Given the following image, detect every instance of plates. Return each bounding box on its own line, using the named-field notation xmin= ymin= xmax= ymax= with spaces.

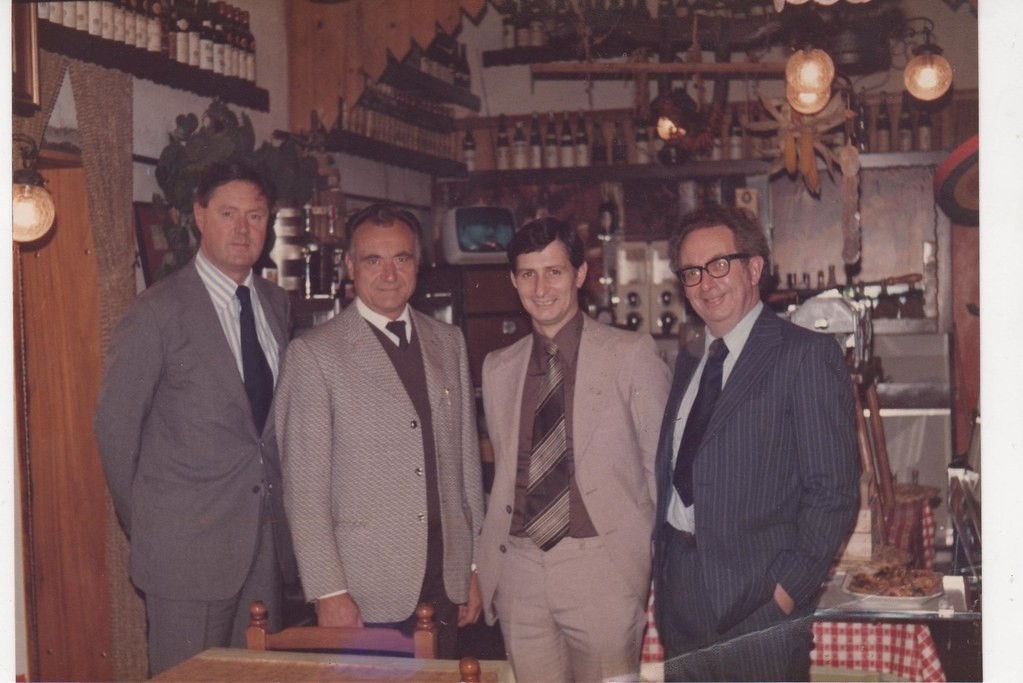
xmin=840 ymin=564 xmax=944 ymax=609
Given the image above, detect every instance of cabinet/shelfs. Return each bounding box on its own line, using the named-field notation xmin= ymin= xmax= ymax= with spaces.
xmin=432 ymin=148 xmax=955 ymax=393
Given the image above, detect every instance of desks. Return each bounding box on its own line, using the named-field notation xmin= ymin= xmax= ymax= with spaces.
xmin=152 ymin=646 xmax=516 ymax=683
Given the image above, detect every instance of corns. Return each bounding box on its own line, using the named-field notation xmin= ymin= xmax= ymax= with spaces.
xmin=782 ymin=126 xmax=821 ymax=194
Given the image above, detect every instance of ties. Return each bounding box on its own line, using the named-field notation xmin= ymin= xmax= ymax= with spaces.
xmin=235 ymin=286 xmax=276 ymax=435
xmin=385 ymin=321 xmax=409 ymax=352
xmin=673 ymin=338 xmax=729 ymax=508
xmin=522 ymin=342 xmax=570 ymax=552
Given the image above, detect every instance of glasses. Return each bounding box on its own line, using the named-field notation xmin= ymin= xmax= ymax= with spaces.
xmin=676 ymin=252 xmax=767 ymax=288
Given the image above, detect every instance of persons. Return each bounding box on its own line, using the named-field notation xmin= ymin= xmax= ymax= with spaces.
xmin=93 ymin=161 xmax=298 ymax=679
xmin=650 ymin=203 xmax=861 ymax=682
xmin=479 ymin=216 xmax=673 ymax=683
xmin=274 ymin=203 xmax=486 ymax=657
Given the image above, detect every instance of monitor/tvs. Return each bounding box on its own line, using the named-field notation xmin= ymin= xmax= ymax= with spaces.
xmin=443 ymin=206 xmax=520 ymax=266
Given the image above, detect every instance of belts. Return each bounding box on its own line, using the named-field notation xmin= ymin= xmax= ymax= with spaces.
xmin=668 ymin=521 xmax=696 ymax=546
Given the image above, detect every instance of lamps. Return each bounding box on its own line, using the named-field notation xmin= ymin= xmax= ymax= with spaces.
xmin=12 ymin=133 xmax=55 ymax=243
xmin=773 ymin=1 xmax=953 ymax=114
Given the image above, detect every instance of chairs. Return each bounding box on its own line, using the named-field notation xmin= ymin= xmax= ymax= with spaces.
xmin=241 ymin=600 xmax=439 ymax=656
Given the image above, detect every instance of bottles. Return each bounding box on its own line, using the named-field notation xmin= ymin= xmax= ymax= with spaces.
xmin=598 ymin=175 xmax=620 ymax=251
xmin=770 ymin=265 xmax=927 ymax=320
xmin=896 ymin=90 xmax=913 ymax=151
xmin=513 ymin=120 xmax=527 ymax=170
xmin=299 ymin=202 xmax=322 ymax=300
xmin=876 ymin=91 xmax=890 ymax=153
xmin=495 ymin=114 xmax=510 ymax=169
xmin=321 ymin=203 xmax=345 ymax=298
xmin=462 ymin=125 xmax=476 ymax=173
xmin=854 ymin=100 xmax=868 ymax=153
xmin=528 ymin=103 xmax=780 ymax=171
xmin=918 ymin=102 xmax=931 ymax=150
xmin=36 ymin=0 xmax=255 ymax=82
